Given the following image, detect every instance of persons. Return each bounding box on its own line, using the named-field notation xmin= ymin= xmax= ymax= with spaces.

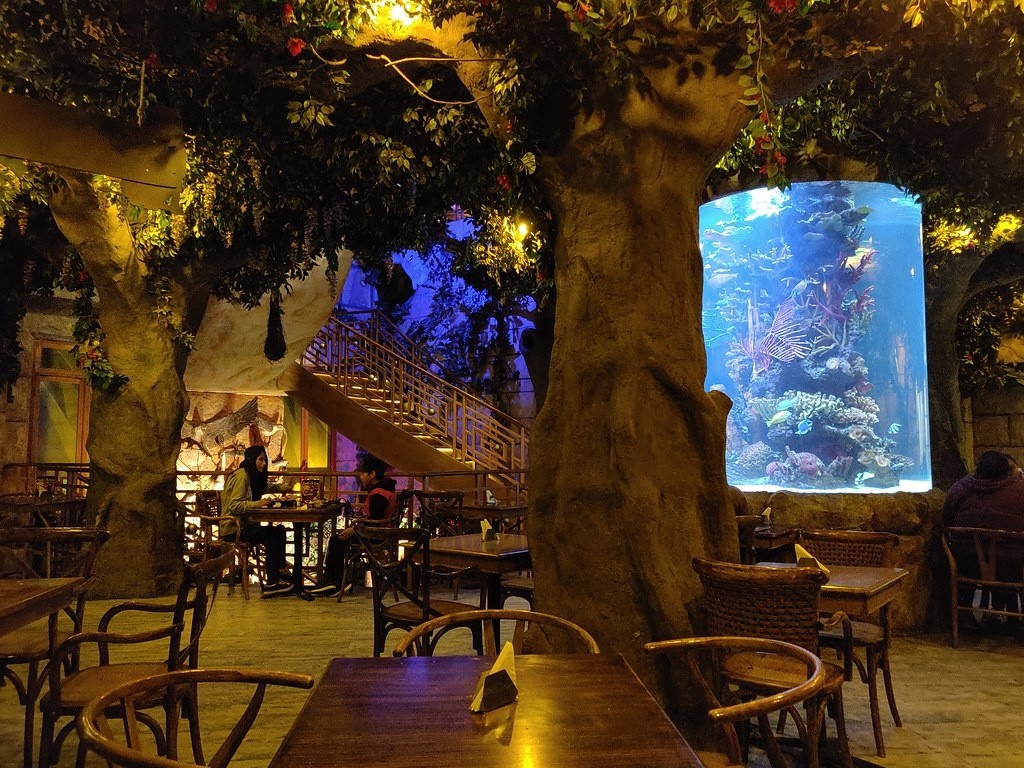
xmin=310 ymin=456 xmax=401 ymax=598
xmin=219 ymin=444 xmax=295 ymax=596
xmin=938 ymin=450 xmax=1024 ymax=644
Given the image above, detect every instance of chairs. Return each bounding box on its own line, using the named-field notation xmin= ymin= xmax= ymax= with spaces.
xmin=500 ymin=576 xmax=535 ymax=611
xmin=796 ymin=530 xmax=902 ymax=758
xmin=691 ymin=556 xmax=854 ymax=768
xmin=0 ymin=527 xmax=111 ymax=768
xmin=38 ymin=542 xmax=235 ymax=768
xmin=644 ymin=636 xmax=827 ymax=768
xmin=736 ymin=515 xmax=762 ymax=565
xmin=338 ymin=490 xmax=408 ymax=602
xmin=76 ymin=667 xmax=313 ymax=768
xmin=941 ymin=526 xmax=1024 ymax=648
xmin=196 ymin=491 xmax=264 ymax=600
xmin=354 ymin=523 xmax=484 ymax=656
xmin=414 ymin=489 xmax=465 ymax=599
xmin=393 ymin=609 xmax=600 ymax=657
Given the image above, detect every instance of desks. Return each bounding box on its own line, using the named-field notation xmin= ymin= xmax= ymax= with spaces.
xmin=753 ymin=562 xmax=910 ymax=768
xmin=247 ymin=500 xmax=344 ymax=601
xmin=398 ymin=533 xmax=532 ymax=655
xmin=268 ymin=655 xmax=707 ymax=768
xmin=0 ymin=497 xmax=86 ymax=578
xmin=0 ymin=578 xmax=90 ymax=674
xmin=453 ymin=506 xmax=528 ymax=535
xmin=739 ymin=524 xmax=800 ymax=565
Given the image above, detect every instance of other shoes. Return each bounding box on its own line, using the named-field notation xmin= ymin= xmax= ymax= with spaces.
xmin=959 ymin=614 xmax=980 ymax=631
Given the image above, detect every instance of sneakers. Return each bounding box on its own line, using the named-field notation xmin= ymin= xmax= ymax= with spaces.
xmin=311 ymin=578 xmax=336 ymax=593
xmin=264 ymin=581 xmax=294 ymax=595
xmin=329 ymin=581 xmax=352 ymax=596
xmin=279 ymin=572 xmax=294 ymax=582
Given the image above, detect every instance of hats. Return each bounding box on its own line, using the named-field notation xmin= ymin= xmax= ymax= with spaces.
xmin=358 ymin=455 xmax=386 ymax=471
